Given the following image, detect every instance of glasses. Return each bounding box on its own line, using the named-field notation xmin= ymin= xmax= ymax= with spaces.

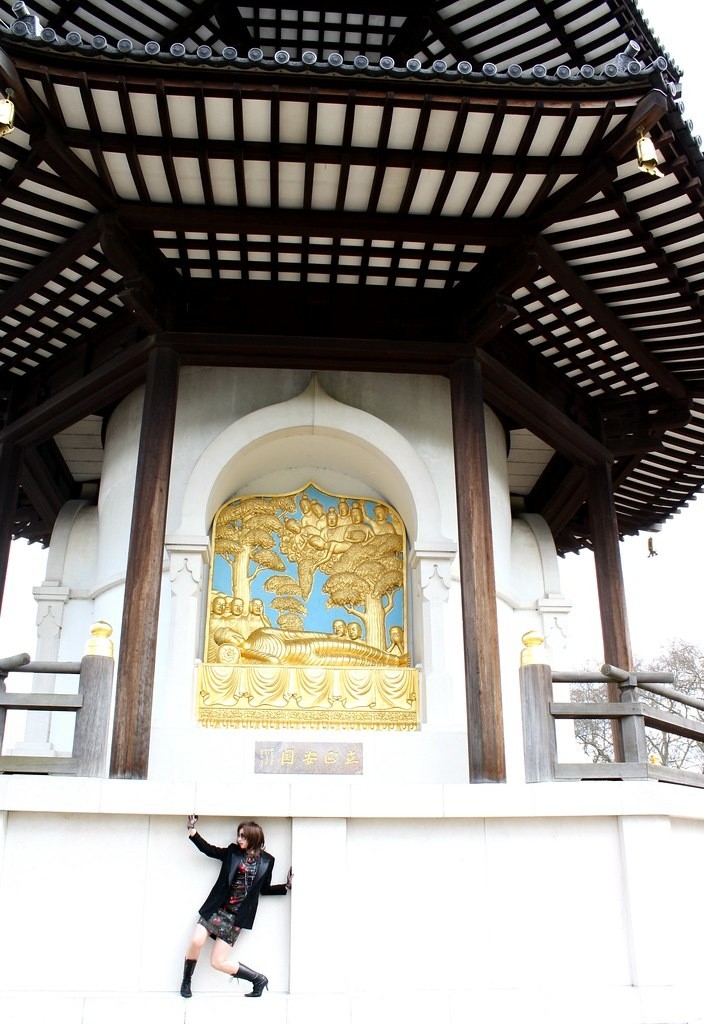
xmin=237 ymin=834 xmax=248 ymax=840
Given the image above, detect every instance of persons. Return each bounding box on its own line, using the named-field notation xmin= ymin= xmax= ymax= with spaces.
xmin=180 ymin=815 xmax=293 ymax=998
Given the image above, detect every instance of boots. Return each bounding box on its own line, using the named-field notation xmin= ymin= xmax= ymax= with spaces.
xmin=230 ymin=961 xmax=268 ymax=997
xmin=180 ymin=957 xmax=197 ymax=998
